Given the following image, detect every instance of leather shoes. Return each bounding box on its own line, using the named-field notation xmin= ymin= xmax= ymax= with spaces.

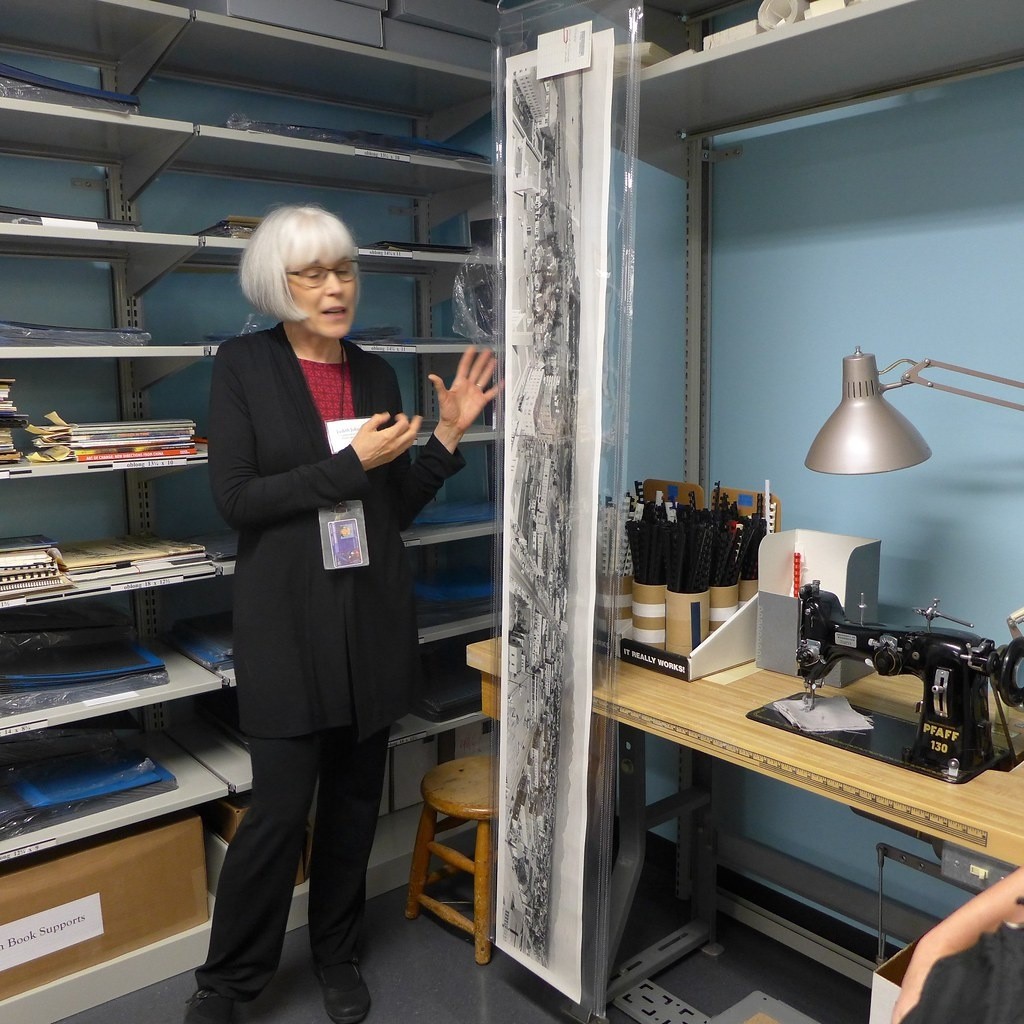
xmin=317 ymin=962 xmax=371 ymax=1024
xmin=184 ymin=989 xmax=235 ymax=1024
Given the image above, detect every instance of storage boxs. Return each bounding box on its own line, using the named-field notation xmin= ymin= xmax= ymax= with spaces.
xmin=757 ymin=528 xmax=881 ymax=688
xmin=0 ymin=809 xmax=209 ymax=1001
xmin=869 ymin=936 xmax=919 ymax=1024
xmin=619 ymin=479 xmax=782 ymax=682
xmin=211 ymin=718 xmax=493 ymax=886
xmin=178 ymin=0 xmax=523 ymax=76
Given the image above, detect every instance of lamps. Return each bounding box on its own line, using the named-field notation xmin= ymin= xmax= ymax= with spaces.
xmin=804 ymin=346 xmax=1024 ymax=475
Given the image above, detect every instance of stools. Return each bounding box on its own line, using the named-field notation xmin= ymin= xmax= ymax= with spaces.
xmin=405 ymin=754 xmax=499 ymax=964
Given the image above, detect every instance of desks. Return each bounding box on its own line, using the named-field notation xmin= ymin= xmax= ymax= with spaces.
xmin=468 ymin=635 xmax=1024 ymax=1024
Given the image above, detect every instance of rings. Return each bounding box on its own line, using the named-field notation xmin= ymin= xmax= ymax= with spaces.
xmin=477 ymin=384 xmax=484 ymax=390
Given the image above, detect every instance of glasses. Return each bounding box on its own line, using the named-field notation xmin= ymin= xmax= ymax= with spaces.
xmin=286 ymin=259 xmax=360 ymax=288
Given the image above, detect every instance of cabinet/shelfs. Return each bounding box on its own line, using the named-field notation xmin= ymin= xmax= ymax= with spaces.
xmin=0 ymin=0 xmax=515 ymax=1024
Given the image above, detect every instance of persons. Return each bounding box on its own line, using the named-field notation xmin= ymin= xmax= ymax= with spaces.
xmin=184 ymin=205 xmax=502 ymax=1024
xmin=890 ymin=865 xmax=1024 ymax=1024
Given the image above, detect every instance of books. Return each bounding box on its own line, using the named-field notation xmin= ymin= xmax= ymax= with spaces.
xmin=0 ymin=207 xmax=262 ymax=599
